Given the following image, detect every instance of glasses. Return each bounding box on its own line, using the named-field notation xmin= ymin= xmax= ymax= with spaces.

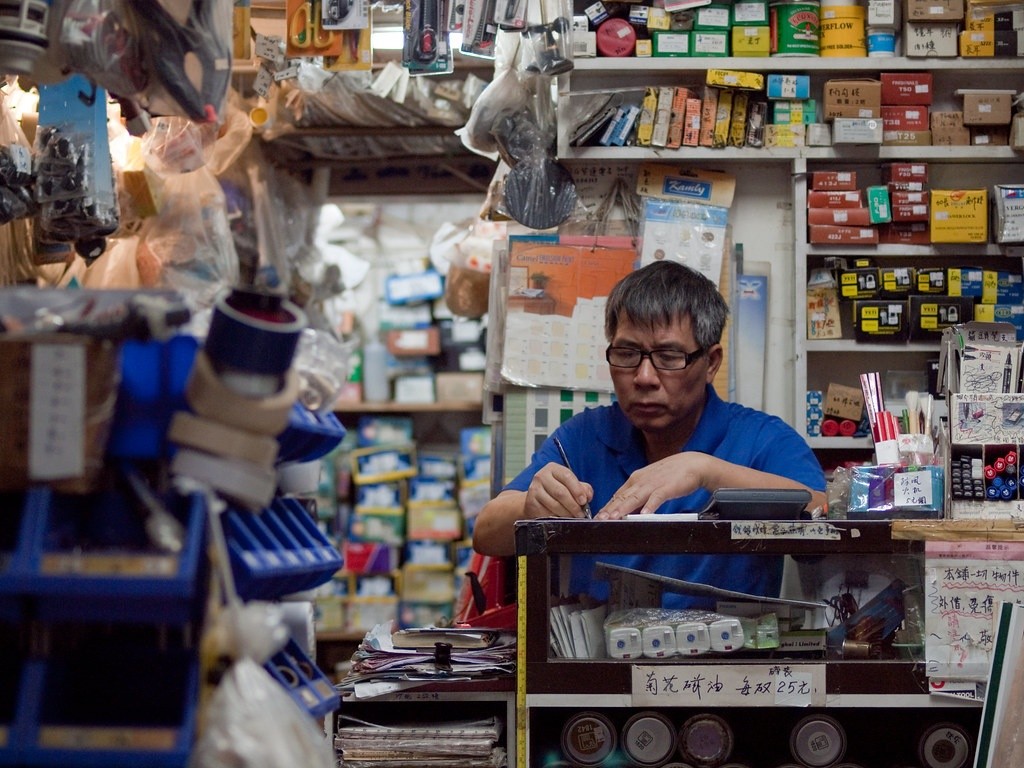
xmin=606 ymin=343 xmax=705 ymax=370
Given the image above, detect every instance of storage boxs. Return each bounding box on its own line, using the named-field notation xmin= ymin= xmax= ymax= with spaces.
xmin=731 ymin=0 xmax=770 ymax=26
xmin=691 ymin=31 xmax=728 ymax=57
xmin=636 ymin=40 xmax=653 ymax=57
xmin=647 ymin=7 xmax=672 ymax=38
xmin=732 ymin=26 xmax=770 ymax=57
xmin=806 ymin=258 xmax=1024 ymax=520
xmin=807 ymin=163 xmax=1024 ymax=245
xmin=584 ymin=1 xmax=628 ymax=26
xmin=327 ymin=249 xmax=490 ymax=405
xmin=283 ymin=416 xmax=493 ymax=635
xmin=629 ymin=5 xmax=649 ymax=25
xmin=573 ymin=14 xmax=588 ymax=32
xmin=867 ymin=0 xmax=1024 ymax=59
xmin=574 ymin=31 xmax=597 ymax=58
xmin=653 ymin=31 xmax=691 ymax=57
xmin=694 ymin=4 xmax=732 ymax=30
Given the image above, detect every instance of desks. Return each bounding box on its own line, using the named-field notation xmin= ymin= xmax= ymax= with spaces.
xmin=510 ymin=517 xmax=1024 ymax=768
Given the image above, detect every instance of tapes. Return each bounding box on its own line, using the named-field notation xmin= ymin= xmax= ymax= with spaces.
xmin=166 ymin=409 xmax=280 ymax=465
xmin=187 ymin=348 xmax=301 ymax=431
xmin=205 ymin=295 xmax=308 ymax=376
xmin=172 ymin=446 xmax=277 ymax=509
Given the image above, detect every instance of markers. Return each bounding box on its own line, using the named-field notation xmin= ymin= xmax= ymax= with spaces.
xmin=984 ymin=451 xmax=1024 ymax=500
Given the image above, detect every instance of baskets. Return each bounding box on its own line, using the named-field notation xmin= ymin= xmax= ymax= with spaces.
xmin=0 ymin=332 xmax=122 ymax=493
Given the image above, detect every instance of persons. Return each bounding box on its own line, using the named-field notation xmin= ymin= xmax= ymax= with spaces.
xmin=473 ymin=261 xmax=829 ymax=614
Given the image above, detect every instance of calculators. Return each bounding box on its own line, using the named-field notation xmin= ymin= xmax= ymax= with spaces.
xmin=698 ymin=487 xmax=812 ymax=519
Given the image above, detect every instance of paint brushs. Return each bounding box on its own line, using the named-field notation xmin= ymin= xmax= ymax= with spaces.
xmin=904 ymin=390 xmax=933 ymax=436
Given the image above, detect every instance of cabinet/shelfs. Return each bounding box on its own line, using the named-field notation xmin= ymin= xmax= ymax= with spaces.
xmin=556 ymin=56 xmax=1024 ymax=450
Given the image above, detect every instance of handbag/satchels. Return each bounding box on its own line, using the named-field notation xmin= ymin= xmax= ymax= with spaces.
xmin=191 ymin=599 xmax=335 ymax=768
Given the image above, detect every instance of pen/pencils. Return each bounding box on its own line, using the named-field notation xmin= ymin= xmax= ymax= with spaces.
xmin=962 ymin=403 xmax=970 ymax=421
xmin=554 ymin=437 xmax=594 ymax=520
xmin=1002 ymin=350 xmax=1012 ymax=393
